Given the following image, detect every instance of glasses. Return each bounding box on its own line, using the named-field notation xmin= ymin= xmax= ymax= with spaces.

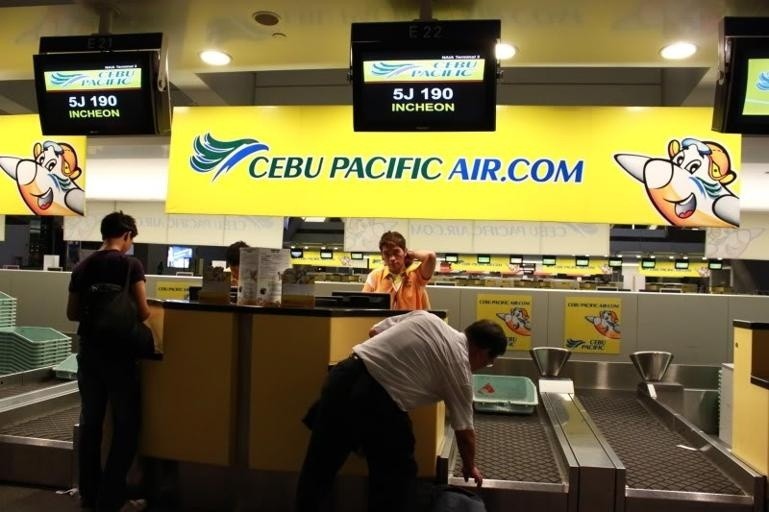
xmin=483 ymin=361 xmax=495 ymax=367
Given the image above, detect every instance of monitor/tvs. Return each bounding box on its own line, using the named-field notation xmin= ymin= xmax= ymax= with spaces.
xmin=166 ymin=245 xmax=196 ymax=273
xmin=351 ymin=37 xmax=496 ymax=132
xmin=711 ymin=36 xmax=769 ymax=136
xmin=332 ymin=292 xmax=391 ymax=310
xmin=32 ymin=51 xmax=170 ymax=135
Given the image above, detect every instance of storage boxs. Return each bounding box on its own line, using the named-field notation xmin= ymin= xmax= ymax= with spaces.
xmin=237 ymin=248 xmax=290 ymax=305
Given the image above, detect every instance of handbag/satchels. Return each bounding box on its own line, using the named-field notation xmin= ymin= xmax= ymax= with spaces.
xmin=104 ymin=255 xmax=138 ymax=335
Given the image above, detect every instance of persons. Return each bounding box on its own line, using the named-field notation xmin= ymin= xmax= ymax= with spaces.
xmin=223 ymin=239 xmax=252 ymax=288
xmin=65 ymin=211 xmax=156 ymax=509
xmin=362 ymin=233 xmax=436 ymax=312
xmin=285 ymin=309 xmax=506 ymax=511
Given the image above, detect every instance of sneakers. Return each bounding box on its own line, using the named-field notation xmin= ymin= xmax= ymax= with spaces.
xmin=120 ymin=498 xmax=147 ymax=512
